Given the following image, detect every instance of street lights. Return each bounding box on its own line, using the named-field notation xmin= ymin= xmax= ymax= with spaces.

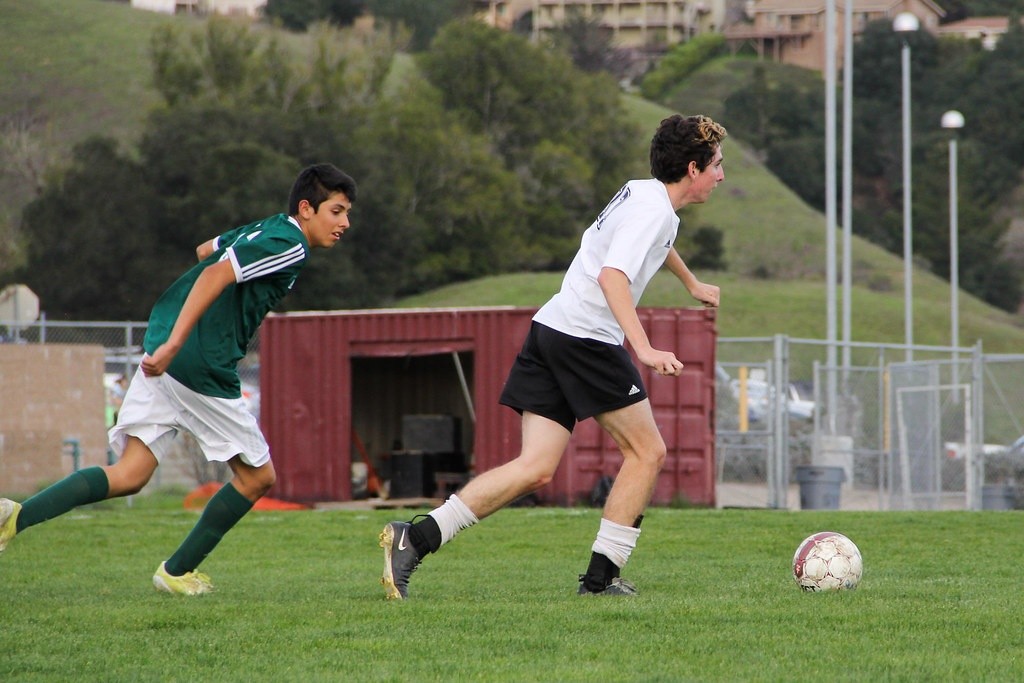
xmin=889 ymin=8 xmax=922 ymax=360
xmin=941 ymin=109 xmax=968 ymax=349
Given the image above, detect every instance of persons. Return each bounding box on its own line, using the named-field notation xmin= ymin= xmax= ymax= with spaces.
xmin=2 ymin=162 xmax=359 ymax=599
xmin=378 ymin=112 xmax=730 ymax=605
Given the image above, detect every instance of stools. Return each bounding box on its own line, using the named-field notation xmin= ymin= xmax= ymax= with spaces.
xmin=435 ymin=472 xmax=467 ymax=496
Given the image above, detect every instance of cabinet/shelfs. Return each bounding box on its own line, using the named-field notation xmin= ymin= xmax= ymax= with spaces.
xmin=400 ymin=414 xmax=464 ymax=453
xmin=389 ymin=450 xmax=449 ymax=498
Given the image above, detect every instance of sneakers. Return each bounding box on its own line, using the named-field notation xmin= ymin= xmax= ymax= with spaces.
xmin=152 ymin=560 xmax=214 ymax=596
xmin=0 ymin=497 xmax=23 ymax=551
xmin=576 ymin=577 xmax=641 ymax=596
xmin=378 ymin=521 xmax=423 ymax=600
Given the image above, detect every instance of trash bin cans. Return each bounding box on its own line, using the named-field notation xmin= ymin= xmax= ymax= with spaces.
xmin=792 ymin=464 xmax=848 ymax=510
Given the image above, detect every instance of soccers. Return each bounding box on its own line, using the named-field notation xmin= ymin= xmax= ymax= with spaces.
xmin=792 ymin=531 xmax=864 ymax=593
